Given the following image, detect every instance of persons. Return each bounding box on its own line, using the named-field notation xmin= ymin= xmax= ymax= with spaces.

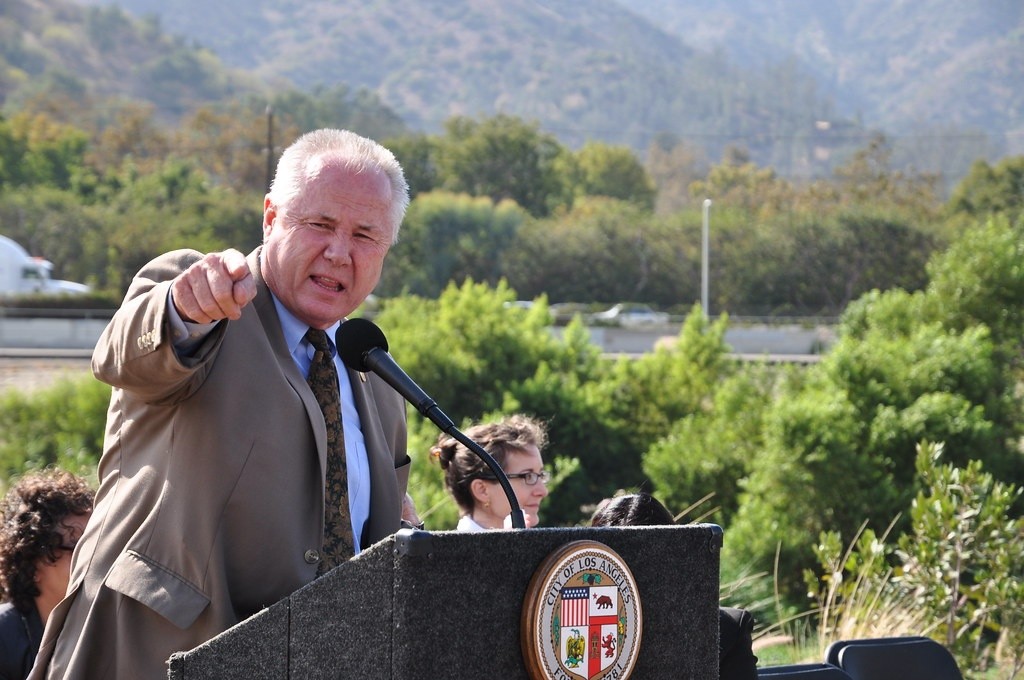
xmin=401 ymin=491 xmax=425 ymax=531
xmin=27 ymin=128 xmax=412 ymax=680
xmin=590 ymin=483 xmax=759 ymax=680
xmin=429 ymin=410 xmax=551 ymax=532
xmin=1 ymin=462 xmax=96 ymax=680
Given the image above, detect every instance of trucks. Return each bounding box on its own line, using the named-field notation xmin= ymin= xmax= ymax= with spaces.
xmin=0 ymin=231 xmax=92 ymax=300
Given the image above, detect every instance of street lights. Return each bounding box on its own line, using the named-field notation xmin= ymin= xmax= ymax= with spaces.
xmin=699 ymin=196 xmax=712 ymax=319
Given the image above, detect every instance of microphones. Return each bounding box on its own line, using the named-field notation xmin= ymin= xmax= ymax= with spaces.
xmin=334 ymin=318 xmax=528 ymax=527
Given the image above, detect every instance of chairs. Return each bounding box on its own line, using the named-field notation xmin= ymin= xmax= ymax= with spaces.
xmin=756 ymin=634 xmax=962 ymax=680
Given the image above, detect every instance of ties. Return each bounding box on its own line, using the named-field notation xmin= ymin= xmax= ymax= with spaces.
xmin=304 ymin=327 xmax=355 ymax=581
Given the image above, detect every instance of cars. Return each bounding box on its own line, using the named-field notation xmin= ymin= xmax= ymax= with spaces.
xmin=548 ymin=301 xmax=590 ymax=325
xmin=591 ymin=300 xmax=670 ymax=329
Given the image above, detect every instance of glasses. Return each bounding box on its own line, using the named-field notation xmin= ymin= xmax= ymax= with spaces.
xmin=481 ymin=469 xmax=551 ymax=485
